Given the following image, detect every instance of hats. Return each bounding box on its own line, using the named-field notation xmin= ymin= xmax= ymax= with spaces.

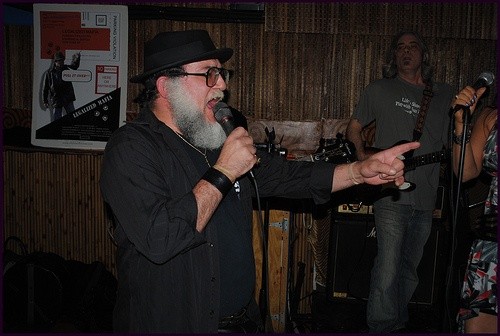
xmin=129 ymin=28 xmax=234 ymax=83
xmin=52 ymin=52 xmax=65 ymax=62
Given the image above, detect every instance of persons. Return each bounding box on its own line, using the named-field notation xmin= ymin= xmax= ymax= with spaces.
xmin=449 ymin=86 xmax=500 ymax=336
xmin=346 ymin=30 xmax=454 ymax=336
xmin=99 ymin=30 xmax=421 ymax=336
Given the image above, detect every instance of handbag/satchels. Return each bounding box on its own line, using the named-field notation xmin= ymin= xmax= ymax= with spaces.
xmin=5 ymin=236 xmax=117 ymax=336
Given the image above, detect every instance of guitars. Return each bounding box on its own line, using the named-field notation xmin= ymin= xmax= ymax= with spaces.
xmin=350 ymin=140 xmax=451 ymax=205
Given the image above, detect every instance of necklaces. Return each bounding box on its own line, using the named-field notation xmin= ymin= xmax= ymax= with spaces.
xmin=171 ymin=126 xmax=209 ymax=166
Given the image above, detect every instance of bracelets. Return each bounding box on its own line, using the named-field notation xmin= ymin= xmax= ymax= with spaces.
xmin=453 ymin=130 xmax=471 ymax=145
xmin=348 ymin=160 xmax=365 ymax=185
xmin=200 ymin=167 xmax=233 ymax=197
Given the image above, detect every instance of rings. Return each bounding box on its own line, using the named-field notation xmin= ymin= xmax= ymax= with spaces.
xmin=455 ymin=94 xmax=459 ymax=99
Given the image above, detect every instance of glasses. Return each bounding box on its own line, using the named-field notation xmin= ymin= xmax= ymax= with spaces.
xmin=174 ymin=66 xmax=235 ymax=87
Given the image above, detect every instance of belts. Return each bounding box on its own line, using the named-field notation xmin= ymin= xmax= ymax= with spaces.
xmin=218 ymin=306 xmax=247 ymax=328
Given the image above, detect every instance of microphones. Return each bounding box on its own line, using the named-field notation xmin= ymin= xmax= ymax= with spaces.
xmin=452 ymin=71 xmax=496 ymax=113
xmin=212 ymin=102 xmax=255 ymax=178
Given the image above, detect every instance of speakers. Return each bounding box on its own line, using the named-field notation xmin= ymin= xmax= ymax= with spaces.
xmin=326 ymin=215 xmax=469 ymax=336
